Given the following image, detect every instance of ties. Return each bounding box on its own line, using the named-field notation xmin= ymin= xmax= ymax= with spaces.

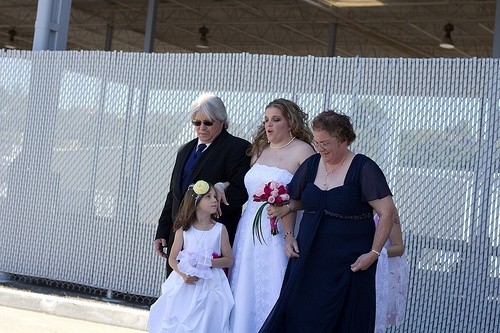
xmin=193 ymin=144 xmax=207 ymax=160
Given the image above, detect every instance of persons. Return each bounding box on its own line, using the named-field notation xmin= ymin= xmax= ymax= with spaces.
xmin=155 ymin=92 xmax=252 ymax=278
xmin=374 ymin=206 xmax=408 ymax=333
xmin=258 ymin=111 xmax=394 ymax=333
xmin=148 ymin=181 xmax=235 ymax=333
xmin=214 ymin=98 xmax=317 ymax=333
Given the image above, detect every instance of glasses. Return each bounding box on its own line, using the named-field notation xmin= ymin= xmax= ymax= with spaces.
xmin=313 ymin=137 xmax=340 ymax=148
xmin=191 ymin=119 xmax=214 ymax=126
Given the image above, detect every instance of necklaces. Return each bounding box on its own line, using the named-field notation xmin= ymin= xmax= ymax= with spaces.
xmin=324 ymin=150 xmax=349 ymax=186
xmin=270 ymin=137 xmax=295 ymax=149
xmin=194 ymin=232 xmax=204 ymax=243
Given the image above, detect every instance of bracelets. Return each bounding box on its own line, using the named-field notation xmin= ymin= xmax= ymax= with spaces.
xmin=284 ymin=232 xmax=291 ymax=239
xmin=287 ymin=204 xmax=291 ymax=211
xmin=372 ymin=250 xmax=379 ymax=255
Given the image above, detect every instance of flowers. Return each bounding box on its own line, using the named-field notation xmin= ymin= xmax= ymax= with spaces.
xmin=253 ymin=181 xmax=290 ymax=246
xmin=193 ymin=180 xmax=209 ymax=194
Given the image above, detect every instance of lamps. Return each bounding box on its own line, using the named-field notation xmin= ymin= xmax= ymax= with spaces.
xmin=197 ymin=26 xmax=209 ymax=48
xmin=439 ymin=23 xmax=456 ymax=49
xmin=5 ymin=27 xmax=17 ymax=48
